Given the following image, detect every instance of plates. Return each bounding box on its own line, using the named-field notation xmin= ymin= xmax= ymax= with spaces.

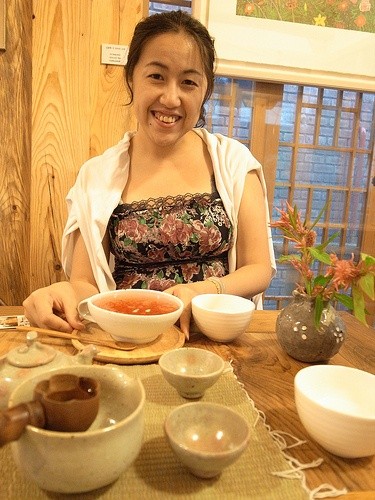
xmin=70 ymin=319 xmax=185 ymax=364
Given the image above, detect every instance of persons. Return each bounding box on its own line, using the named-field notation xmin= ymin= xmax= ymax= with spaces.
xmin=22 ymin=9 xmax=276 ymax=341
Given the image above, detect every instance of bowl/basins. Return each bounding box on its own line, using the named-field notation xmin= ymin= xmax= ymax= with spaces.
xmin=191 ymin=293 xmax=255 ymax=343
xmin=158 ymin=347 xmax=225 ymax=399
xmin=295 ymin=364 xmax=375 ymax=458
xmin=77 ymin=289 xmax=184 ymax=344
xmin=164 ymin=401 xmax=253 ymax=480
xmin=8 ymin=363 xmax=146 ymax=494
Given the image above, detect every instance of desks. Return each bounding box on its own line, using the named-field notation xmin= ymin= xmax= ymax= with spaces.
xmin=0 ymin=305 xmax=375 ymax=500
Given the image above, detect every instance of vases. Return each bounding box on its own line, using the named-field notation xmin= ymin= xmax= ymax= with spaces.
xmin=276 ymin=290 xmax=345 ymax=362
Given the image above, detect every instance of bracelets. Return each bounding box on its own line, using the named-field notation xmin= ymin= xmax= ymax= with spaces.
xmin=206 ymin=277 xmax=223 ymax=294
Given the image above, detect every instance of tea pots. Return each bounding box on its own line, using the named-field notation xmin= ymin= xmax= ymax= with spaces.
xmin=0 ymin=331 xmax=100 ymax=407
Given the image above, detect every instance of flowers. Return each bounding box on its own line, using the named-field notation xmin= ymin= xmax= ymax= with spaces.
xmin=268 ymin=200 xmax=375 ymax=333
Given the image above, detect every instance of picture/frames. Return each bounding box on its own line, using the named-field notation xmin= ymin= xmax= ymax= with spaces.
xmin=190 ymin=0 xmax=375 ymax=94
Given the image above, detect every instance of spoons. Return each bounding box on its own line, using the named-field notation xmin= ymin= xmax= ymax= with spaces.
xmin=0 ymin=373 xmax=101 ymax=448
xmin=16 ymin=326 xmax=139 ymax=350
